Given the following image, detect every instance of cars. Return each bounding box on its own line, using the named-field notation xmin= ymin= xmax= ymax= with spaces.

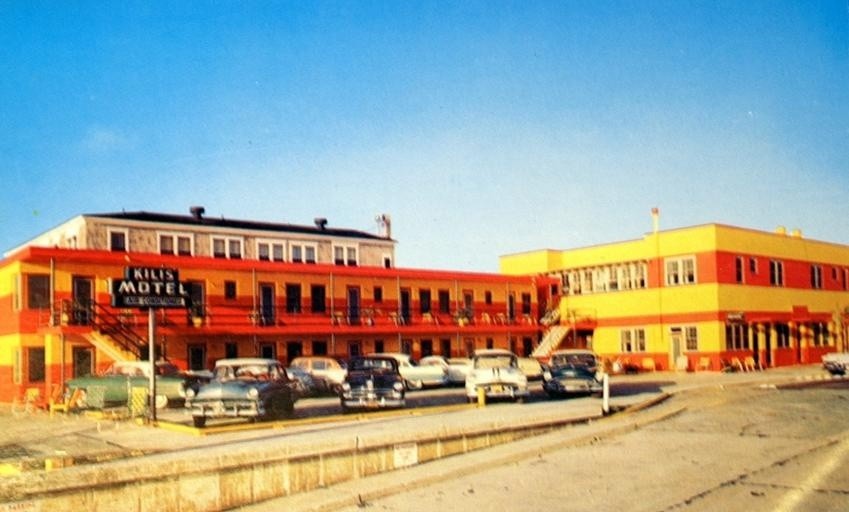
xmin=822 ymin=350 xmax=849 ymax=376
